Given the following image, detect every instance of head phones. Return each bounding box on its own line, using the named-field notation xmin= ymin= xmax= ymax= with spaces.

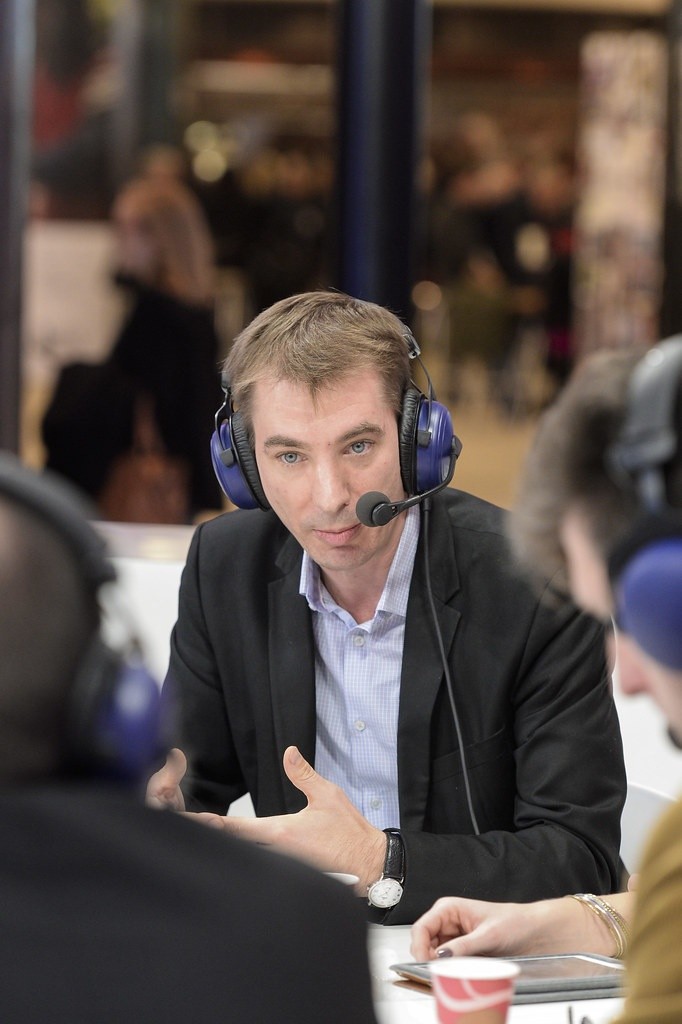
xmin=615 ymin=334 xmax=682 ymax=674
xmin=206 ymin=331 xmax=460 ymax=514
xmin=0 ymin=458 xmax=170 ymax=807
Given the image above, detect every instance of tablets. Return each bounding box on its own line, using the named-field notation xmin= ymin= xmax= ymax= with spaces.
xmin=390 ymin=951 xmax=627 ymax=994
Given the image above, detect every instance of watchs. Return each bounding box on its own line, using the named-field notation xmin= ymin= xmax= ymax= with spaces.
xmin=366 ymin=832 xmax=405 ymax=924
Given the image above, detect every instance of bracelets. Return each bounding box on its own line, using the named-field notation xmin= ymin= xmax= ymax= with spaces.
xmin=566 ymin=894 xmax=633 ymax=959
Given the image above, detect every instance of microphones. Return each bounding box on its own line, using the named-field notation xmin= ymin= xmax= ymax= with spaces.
xmin=355 ymin=454 xmax=457 ymax=528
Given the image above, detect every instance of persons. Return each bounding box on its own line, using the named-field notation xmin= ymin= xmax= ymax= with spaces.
xmin=139 ymin=292 xmax=630 ymax=926
xmin=411 ymin=334 xmax=682 ymax=1024
xmin=444 ymin=105 xmax=571 ymax=286
xmin=208 ymin=118 xmax=330 ymax=309
xmin=96 ymin=172 xmax=232 ymax=525
xmin=0 ymin=447 xmax=376 ymax=1024
xmin=36 ymin=83 xmax=123 ymax=194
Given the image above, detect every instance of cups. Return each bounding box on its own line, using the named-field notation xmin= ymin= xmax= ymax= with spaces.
xmin=321 ymin=873 xmax=360 ymax=893
xmin=430 ymin=956 xmax=520 ymax=1024
